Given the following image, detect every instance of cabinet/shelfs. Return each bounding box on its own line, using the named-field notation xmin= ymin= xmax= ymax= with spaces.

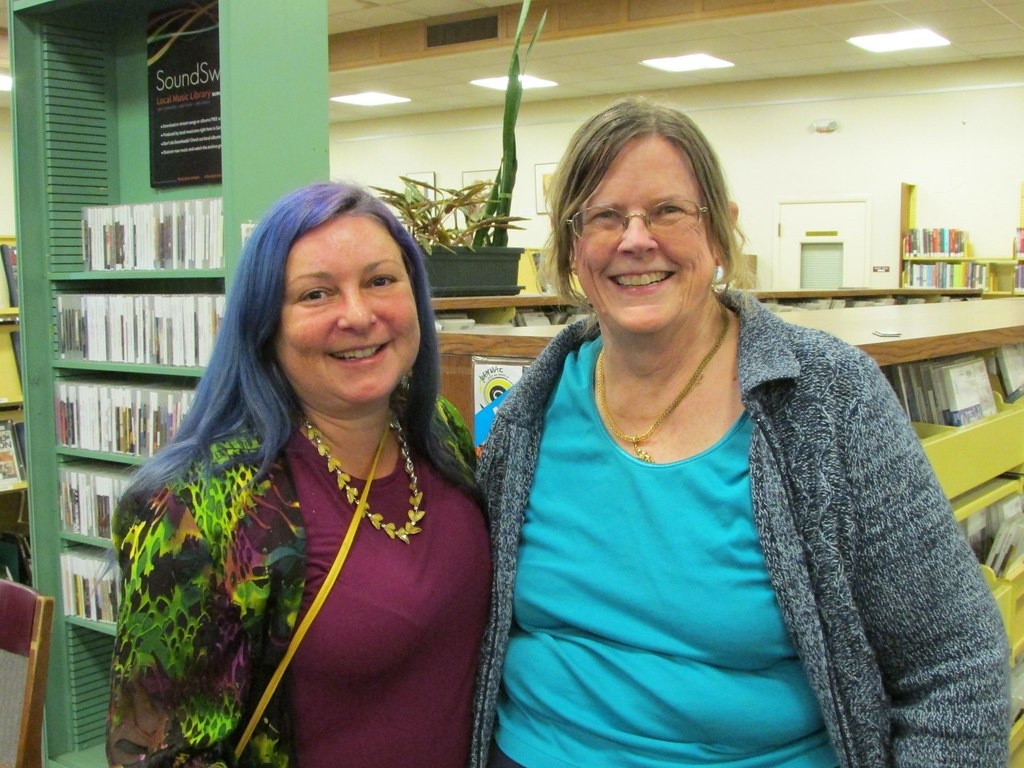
xmin=430 ymin=291 xmax=1021 ymax=767
xmin=10 ymin=1 xmax=329 ymax=768
xmin=897 ymin=183 xmax=1024 ymax=292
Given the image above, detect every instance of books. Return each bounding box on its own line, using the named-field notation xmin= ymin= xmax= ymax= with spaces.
xmin=761 ymin=226 xmax=1024 ymax=729
xmin=0 ymin=194 xmax=225 ymax=630
xmin=430 ymin=303 xmax=594 ymax=329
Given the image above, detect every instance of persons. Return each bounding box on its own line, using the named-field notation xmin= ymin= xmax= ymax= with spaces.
xmin=474 ymin=98 xmax=1014 ymax=768
xmin=104 ymin=185 xmax=490 ymax=768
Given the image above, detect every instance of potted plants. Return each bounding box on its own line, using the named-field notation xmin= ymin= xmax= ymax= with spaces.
xmin=416 ymin=0 xmax=550 ymax=294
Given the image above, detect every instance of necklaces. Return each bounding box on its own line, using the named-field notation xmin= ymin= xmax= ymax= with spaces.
xmin=596 ymin=301 xmax=731 ymax=465
xmin=296 ymin=409 xmax=426 ymax=546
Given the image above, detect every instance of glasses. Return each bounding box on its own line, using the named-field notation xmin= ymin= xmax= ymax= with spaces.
xmin=567 ymin=197 xmax=708 ymax=244
